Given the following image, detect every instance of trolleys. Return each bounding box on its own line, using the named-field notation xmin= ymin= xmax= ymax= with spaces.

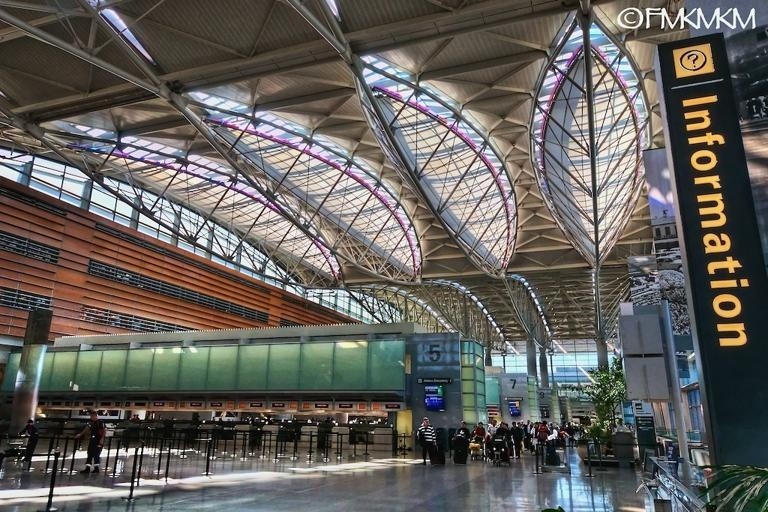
xmin=5 ymin=432 xmax=29 ymax=464
xmin=468 ymin=439 xmax=485 ymax=462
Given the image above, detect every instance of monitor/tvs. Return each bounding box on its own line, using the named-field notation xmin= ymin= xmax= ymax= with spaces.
xmin=540 ymin=406 xmax=549 ymax=418
xmin=424 ymin=384 xmax=446 ymax=412
xmin=508 ymin=400 xmax=521 ymax=417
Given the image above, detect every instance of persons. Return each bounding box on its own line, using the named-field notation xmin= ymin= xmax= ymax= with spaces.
xmin=451 ymin=418 xmax=581 ymax=466
xmin=17 ymin=473 xmax=32 ymax=490
xmin=75 ymin=409 xmax=105 ymax=475
xmin=75 ymin=476 xmax=98 ymax=511
xmin=416 ymin=417 xmax=438 ymax=465
xmin=19 ymin=419 xmax=39 ymax=471
